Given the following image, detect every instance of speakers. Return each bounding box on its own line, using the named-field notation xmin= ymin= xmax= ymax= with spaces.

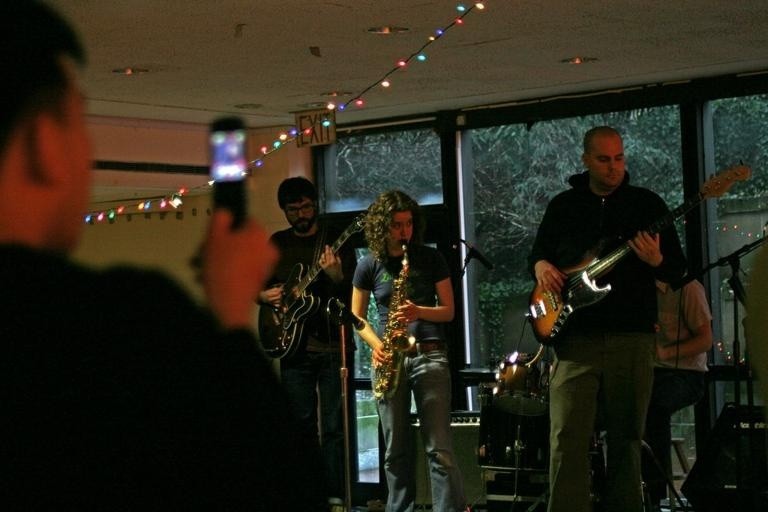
xmin=681 ymin=403 xmax=768 ymax=512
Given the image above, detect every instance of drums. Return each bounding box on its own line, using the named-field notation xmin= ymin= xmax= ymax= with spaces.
xmin=493 ymin=352 xmax=549 ymax=417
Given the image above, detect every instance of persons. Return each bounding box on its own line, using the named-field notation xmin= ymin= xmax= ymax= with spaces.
xmin=527 ymin=125 xmax=687 ymax=511
xmin=0 ymin=1 xmax=338 ymax=511
xmin=348 ymin=187 xmax=467 ymax=511
xmin=254 ymin=175 xmax=357 ymax=506
xmin=641 ymin=275 xmax=714 ymax=498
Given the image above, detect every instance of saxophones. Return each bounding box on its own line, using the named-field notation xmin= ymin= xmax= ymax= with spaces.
xmin=370 ymin=244 xmax=411 ymax=400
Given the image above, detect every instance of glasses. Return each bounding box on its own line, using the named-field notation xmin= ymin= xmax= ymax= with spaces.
xmin=283 ymin=202 xmax=316 ymax=217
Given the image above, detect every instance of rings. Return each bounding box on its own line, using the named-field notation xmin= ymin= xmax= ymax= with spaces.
xmin=322 ymin=260 xmax=326 ymax=264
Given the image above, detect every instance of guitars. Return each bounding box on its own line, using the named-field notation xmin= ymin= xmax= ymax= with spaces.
xmin=259 ymin=214 xmax=367 ymax=359
xmin=529 ymin=165 xmax=751 ymax=343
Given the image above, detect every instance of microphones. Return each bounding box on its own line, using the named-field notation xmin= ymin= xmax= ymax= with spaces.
xmin=462 ymin=240 xmax=493 ymax=271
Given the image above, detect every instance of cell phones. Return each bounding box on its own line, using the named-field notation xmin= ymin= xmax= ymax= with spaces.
xmin=207 ymin=114 xmax=250 ymax=233
